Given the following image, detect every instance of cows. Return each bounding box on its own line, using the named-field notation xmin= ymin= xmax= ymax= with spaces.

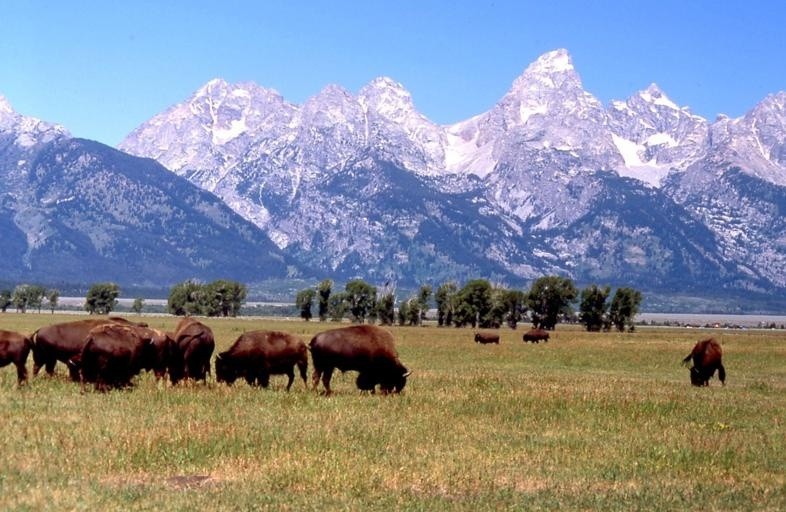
xmin=305 ymin=324 xmax=413 ymax=397
xmin=30 ymin=314 xmax=215 ymax=396
xmin=475 ymin=333 xmax=499 ymax=344
xmin=523 ymin=330 xmax=550 ymax=344
xmin=215 ymin=330 xmax=308 ymax=393
xmin=0 ymin=330 xmax=31 ymax=390
xmin=680 ymin=337 xmax=725 ymax=387
